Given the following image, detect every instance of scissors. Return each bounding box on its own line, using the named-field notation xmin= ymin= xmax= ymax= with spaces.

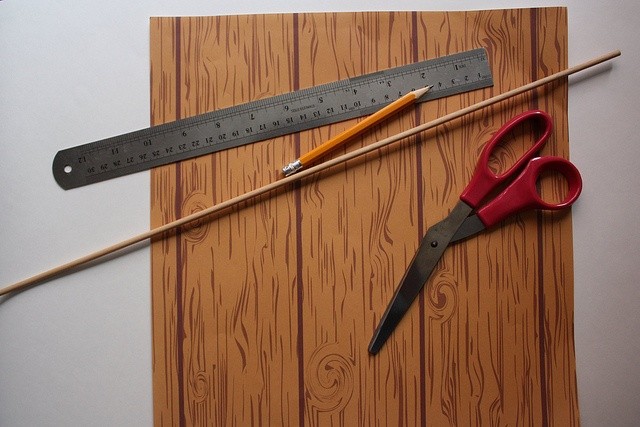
xmin=368 ymin=109 xmax=583 ymax=356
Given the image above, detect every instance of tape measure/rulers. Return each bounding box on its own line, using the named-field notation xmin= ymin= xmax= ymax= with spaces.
xmin=52 ymin=49 xmax=494 ymax=190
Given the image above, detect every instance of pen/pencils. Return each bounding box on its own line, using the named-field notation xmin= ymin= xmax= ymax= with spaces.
xmin=282 ymin=82 xmax=434 ymax=176
